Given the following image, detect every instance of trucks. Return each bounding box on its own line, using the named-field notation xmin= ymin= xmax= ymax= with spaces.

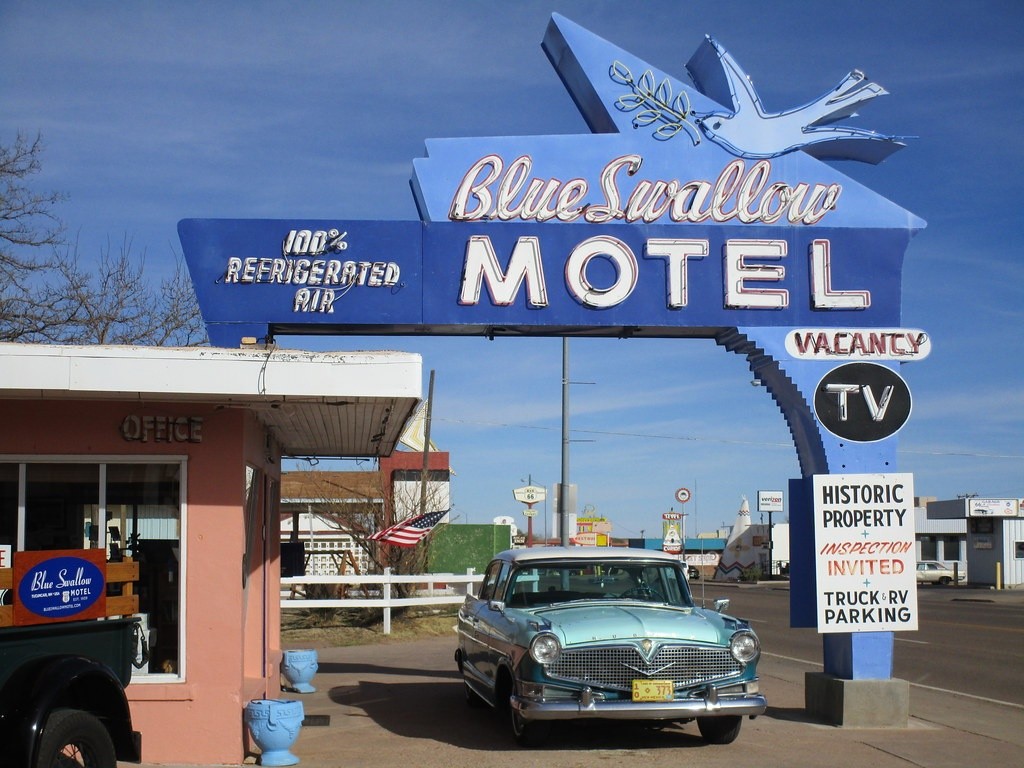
xmin=0 ymin=548 xmax=149 ymax=768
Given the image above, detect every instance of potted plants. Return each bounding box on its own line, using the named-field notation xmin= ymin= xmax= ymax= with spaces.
xmin=244 ymin=699 xmax=306 ymax=767
xmin=281 ymin=649 xmax=319 ymax=694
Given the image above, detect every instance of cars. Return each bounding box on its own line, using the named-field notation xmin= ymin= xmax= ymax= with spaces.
xmin=916 ymin=560 xmax=966 ymax=585
xmin=666 ymin=561 xmax=700 ymax=582
xmin=454 ymin=545 xmax=768 ymax=746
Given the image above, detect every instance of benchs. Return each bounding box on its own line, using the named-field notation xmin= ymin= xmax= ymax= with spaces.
xmin=512 ymin=590 xmax=605 ymax=606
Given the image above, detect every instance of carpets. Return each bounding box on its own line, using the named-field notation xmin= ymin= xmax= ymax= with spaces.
xmin=302 ymin=714 xmax=330 ymax=726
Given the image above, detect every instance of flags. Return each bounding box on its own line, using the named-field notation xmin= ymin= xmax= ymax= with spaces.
xmin=365 ymin=508 xmax=450 ymax=548
